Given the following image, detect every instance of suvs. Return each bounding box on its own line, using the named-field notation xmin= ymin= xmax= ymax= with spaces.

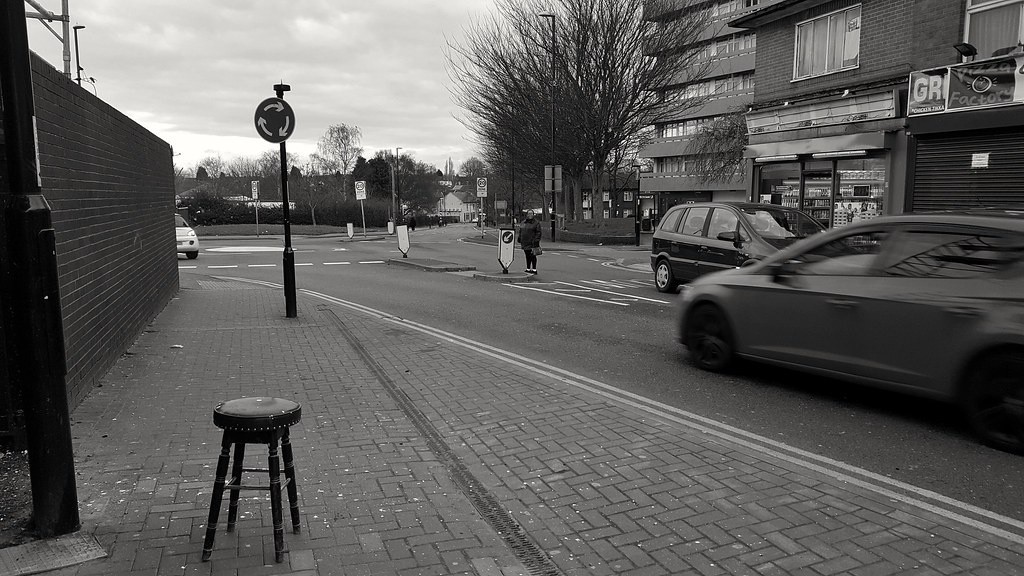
xmin=650 ymin=198 xmax=859 ymax=293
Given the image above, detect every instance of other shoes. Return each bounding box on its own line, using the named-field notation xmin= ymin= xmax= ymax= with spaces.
xmin=528 ymin=270 xmax=538 ymax=276
xmin=522 ymin=270 xmax=531 ymax=273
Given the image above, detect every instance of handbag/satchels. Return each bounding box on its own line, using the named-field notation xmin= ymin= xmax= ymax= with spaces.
xmin=530 ymin=245 xmax=543 ymax=255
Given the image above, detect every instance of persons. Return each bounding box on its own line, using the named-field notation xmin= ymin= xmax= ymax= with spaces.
xmin=389 ymin=213 xmax=443 ymax=235
xmin=842 ymin=202 xmax=876 ymax=225
xmin=517 ymin=209 xmax=541 ymax=275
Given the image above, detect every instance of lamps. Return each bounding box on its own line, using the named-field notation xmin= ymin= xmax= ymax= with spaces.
xmin=953 ymin=43 xmax=978 ymax=57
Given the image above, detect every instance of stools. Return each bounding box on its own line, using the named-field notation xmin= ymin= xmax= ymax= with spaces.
xmin=201 ymin=396 xmax=301 ymax=564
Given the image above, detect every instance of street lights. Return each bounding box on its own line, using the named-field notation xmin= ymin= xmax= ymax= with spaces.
xmin=396 ymin=146 xmax=403 ymax=219
xmin=537 ymin=11 xmax=557 ymax=244
xmin=632 ymin=164 xmax=640 ymax=247
xmin=73 ymin=25 xmax=86 ymax=85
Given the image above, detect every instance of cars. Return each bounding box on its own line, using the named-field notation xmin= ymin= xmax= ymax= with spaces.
xmin=175 ymin=213 xmax=199 ymax=259
xmin=672 ymin=209 xmax=1024 ymax=457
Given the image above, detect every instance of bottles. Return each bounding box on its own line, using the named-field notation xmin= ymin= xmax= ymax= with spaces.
xmin=808 ymin=188 xmax=831 ymax=197
xmin=782 ymin=199 xmax=830 ymax=208
xmin=808 ymin=210 xmax=830 ymax=219
